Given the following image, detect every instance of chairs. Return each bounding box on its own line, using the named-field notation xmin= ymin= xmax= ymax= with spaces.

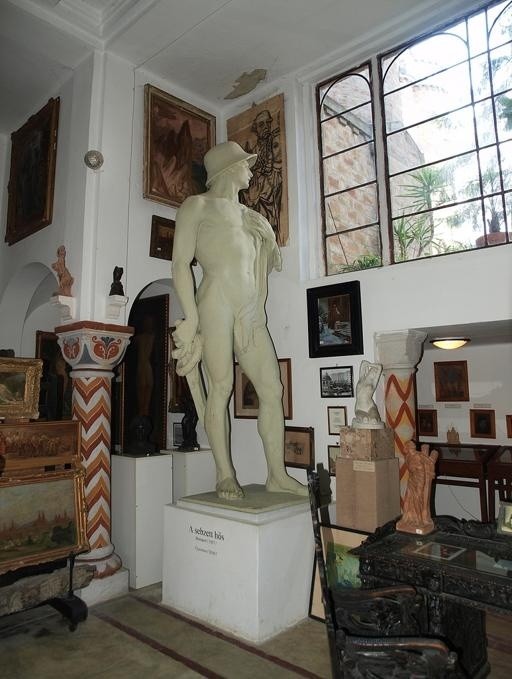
xmin=306 ymin=462 xmax=449 ymax=678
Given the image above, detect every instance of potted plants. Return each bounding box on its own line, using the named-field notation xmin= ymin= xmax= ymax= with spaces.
xmin=450 ymin=165 xmax=511 ymax=247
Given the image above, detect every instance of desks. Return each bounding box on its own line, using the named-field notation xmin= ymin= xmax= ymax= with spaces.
xmin=349 ymin=510 xmax=512 ymax=678
xmin=487 ymin=445 xmax=512 ymax=523
xmin=415 ymin=441 xmax=499 ymax=522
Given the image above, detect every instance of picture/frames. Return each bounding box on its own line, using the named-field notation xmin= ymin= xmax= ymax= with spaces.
xmin=328 ymin=445 xmax=340 ymax=476
xmin=0 ymin=420 xmax=82 ymax=469
xmin=470 ymin=409 xmax=496 ymax=439
xmin=4 ymin=96 xmax=60 ymax=247
xmin=149 ymin=214 xmax=198 ymax=266
xmin=319 ymin=366 xmax=354 ymax=398
xmin=0 ymin=356 xmax=44 ymax=419
xmin=307 ymin=523 xmax=375 ymax=624
xmin=142 ymin=82 xmax=216 ymax=209
xmin=327 ymin=406 xmax=347 ymax=435
xmin=33 ymin=330 xmax=72 ymax=420
xmin=233 ymin=358 xmax=293 ymax=420
xmin=283 ymin=425 xmax=315 ymax=470
xmin=417 ymin=408 xmax=438 ymax=437
xmin=126 ymin=293 xmax=169 ymax=451
xmin=0 ymin=468 xmax=89 ymax=575
xmin=434 ymin=360 xmax=470 ymax=402
xmin=306 ymin=280 xmax=365 ymax=358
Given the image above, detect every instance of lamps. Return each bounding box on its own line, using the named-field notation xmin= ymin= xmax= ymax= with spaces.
xmin=429 ymin=337 xmax=471 ymax=351
